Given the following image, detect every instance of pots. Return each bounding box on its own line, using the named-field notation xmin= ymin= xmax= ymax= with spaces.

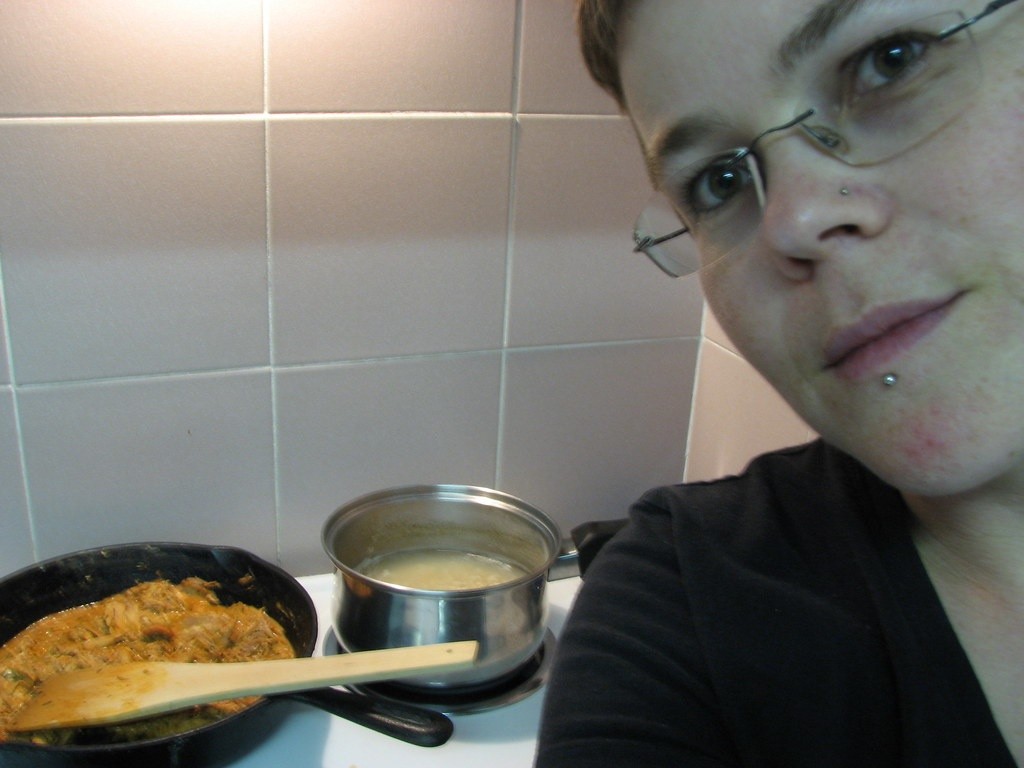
xmin=0 ymin=539 xmax=454 ymax=768
xmin=320 ymin=482 xmax=581 ymax=688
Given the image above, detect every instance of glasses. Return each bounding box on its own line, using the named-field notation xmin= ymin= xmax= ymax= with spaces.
xmin=632 ymin=0 xmax=1013 ymax=279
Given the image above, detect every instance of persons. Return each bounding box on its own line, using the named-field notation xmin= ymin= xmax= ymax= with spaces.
xmin=535 ymin=1 xmax=1024 ymax=768
